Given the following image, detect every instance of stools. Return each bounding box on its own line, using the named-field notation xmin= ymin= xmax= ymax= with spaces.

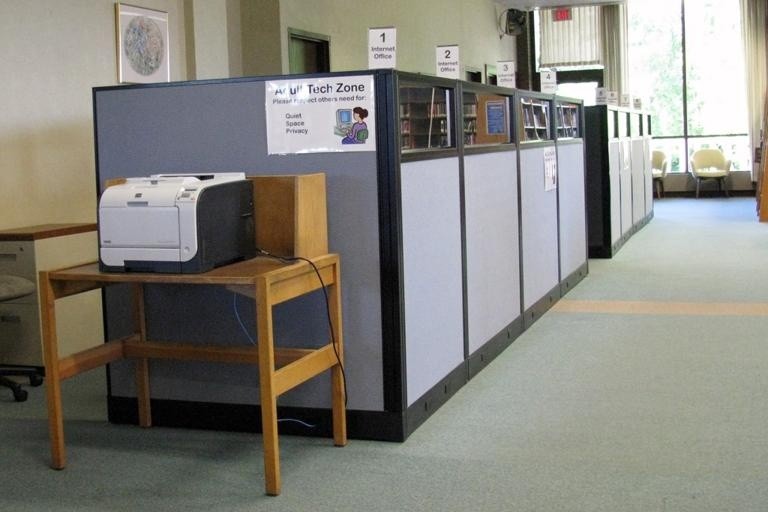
xmin=0 ymin=276 xmax=43 ymax=401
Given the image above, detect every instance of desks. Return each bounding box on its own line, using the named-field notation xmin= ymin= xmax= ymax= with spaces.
xmin=39 ymin=252 xmax=348 ymax=496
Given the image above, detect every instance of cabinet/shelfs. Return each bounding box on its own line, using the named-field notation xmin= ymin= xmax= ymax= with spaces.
xmin=0 ymin=223 xmax=104 ymax=377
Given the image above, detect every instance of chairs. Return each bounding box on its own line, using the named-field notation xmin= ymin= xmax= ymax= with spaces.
xmin=690 ymin=148 xmax=732 ymax=199
xmin=652 ymin=150 xmax=668 ymax=200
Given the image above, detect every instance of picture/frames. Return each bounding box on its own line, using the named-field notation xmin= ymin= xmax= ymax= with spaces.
xmin=115 ymin=2 xmax=170 ymax=84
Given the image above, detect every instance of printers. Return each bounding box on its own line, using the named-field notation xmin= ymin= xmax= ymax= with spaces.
xmin=97 ymin=172 xmax=256 ymax=274
